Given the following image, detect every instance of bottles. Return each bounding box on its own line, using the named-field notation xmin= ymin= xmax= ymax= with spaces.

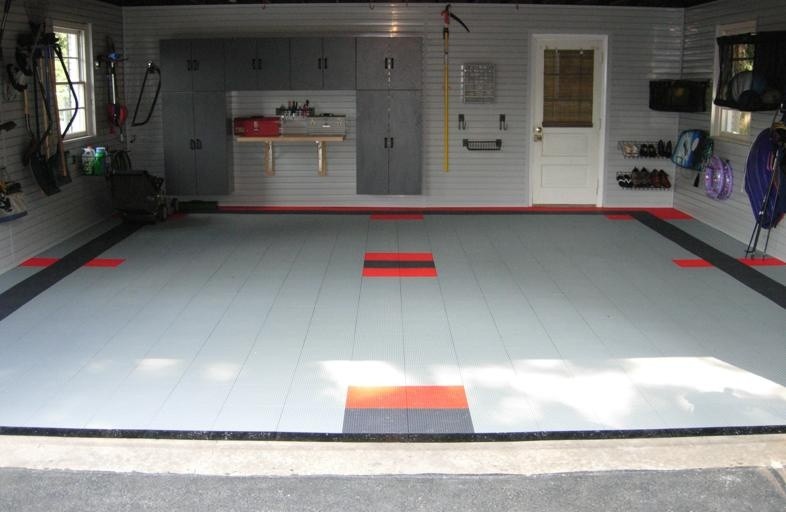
xmin=277 ymin=108 xmax=312 ymax=117
xmin=80 ymin=144 xmax=112 ymax=177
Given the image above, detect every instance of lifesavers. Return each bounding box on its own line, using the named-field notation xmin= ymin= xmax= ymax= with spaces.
xmin=706 ymin=157 xmax=732 ymax=199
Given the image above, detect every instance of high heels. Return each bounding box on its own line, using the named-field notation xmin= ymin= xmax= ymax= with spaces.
xmin=623 ymin=140 xmax=672 ymax=158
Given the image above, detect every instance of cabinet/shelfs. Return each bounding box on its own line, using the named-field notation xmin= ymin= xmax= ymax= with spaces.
xmin=225 ymin=37 xmax=289 ymax=90
xmin=355 ymin=90 xmax=421 ymax=195
xmin=159 ymin=37 xmax=225 ymax=91
xmin=714 ymin=30 xmax=786 ymax=110
xmin=290 ymin=37 xmax=356 ymax=89
xmin=160 ymin=91 xmax=232 ymax=194
xmin=235 ymin=133 xmax=347 ymax=178
xmin=615 ymin=140 xmax=673 ymax=190
xmin=355 ymin=38 xmax=421 ymax=89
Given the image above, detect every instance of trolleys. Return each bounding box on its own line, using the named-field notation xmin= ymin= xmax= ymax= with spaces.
xmin=110 ymin=170 xmax=180 ymax=224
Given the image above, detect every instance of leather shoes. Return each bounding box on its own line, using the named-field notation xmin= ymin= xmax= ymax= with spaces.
xmin=617 ymin=167 xmax=671 ymax=188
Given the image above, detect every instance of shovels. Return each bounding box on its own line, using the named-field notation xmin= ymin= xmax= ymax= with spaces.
xmin=31 ymin=54 xmax=61 ymax=196
xmin=47 ymin=44 xmax=71 ymax=187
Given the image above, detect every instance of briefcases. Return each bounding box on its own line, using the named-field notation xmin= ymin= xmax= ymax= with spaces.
xmin=234 ymin=116 xmax=279 ymax=137
